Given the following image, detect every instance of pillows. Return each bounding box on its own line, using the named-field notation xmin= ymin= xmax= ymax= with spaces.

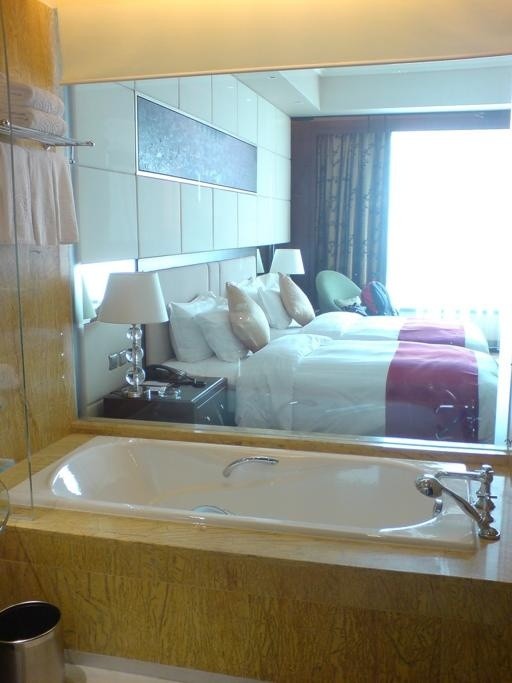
xmin=166 ymin=271 xmax=316 ymax=363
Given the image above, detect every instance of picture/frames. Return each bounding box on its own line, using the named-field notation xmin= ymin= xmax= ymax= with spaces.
xmin=135 ymin=91 xmax=259 ymax=196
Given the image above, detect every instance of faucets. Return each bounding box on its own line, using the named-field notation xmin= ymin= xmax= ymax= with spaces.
xmin=435 ymin=463 xmax=494 ymax=510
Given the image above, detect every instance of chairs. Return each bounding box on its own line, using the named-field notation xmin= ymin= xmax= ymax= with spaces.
xmin=317 ymin=268 xmax=362 ymax=313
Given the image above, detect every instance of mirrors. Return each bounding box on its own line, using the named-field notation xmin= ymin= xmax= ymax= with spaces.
xmin=60 ymin=52 xmax=512 ymax=456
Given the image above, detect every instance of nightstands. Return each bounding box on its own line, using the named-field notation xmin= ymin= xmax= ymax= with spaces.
xmin=104 ymin=375 xmax=229 ymax=425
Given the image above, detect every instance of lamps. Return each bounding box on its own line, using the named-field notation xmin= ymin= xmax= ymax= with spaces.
xmin=97 ymin=273 xmax=170 ymax=397
xmin=269 ymin=248 xmax=305 ymax=276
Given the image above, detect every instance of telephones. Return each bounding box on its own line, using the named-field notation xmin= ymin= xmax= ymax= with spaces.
xmin=145 ymin=365 xmax=186 ymax=383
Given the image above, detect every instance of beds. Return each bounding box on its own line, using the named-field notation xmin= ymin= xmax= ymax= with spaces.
xmin=140 ymin=262 xmax=499 ymax=445
xmin=218 ymin=256 xmax=490 ymax=355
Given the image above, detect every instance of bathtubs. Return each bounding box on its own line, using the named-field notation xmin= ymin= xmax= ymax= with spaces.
xmin=0 ymin=434 xmax=476 ymax=552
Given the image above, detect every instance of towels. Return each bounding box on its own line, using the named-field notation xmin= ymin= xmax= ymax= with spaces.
xmin=0 ymin=75 xmax=67 ymax=138
xmin=0 ymin=143 xmax=79 ymax=247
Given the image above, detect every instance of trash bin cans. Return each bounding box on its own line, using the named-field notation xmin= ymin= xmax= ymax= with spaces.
xmin=0 ymin=600 xmax=63 ymax=683
